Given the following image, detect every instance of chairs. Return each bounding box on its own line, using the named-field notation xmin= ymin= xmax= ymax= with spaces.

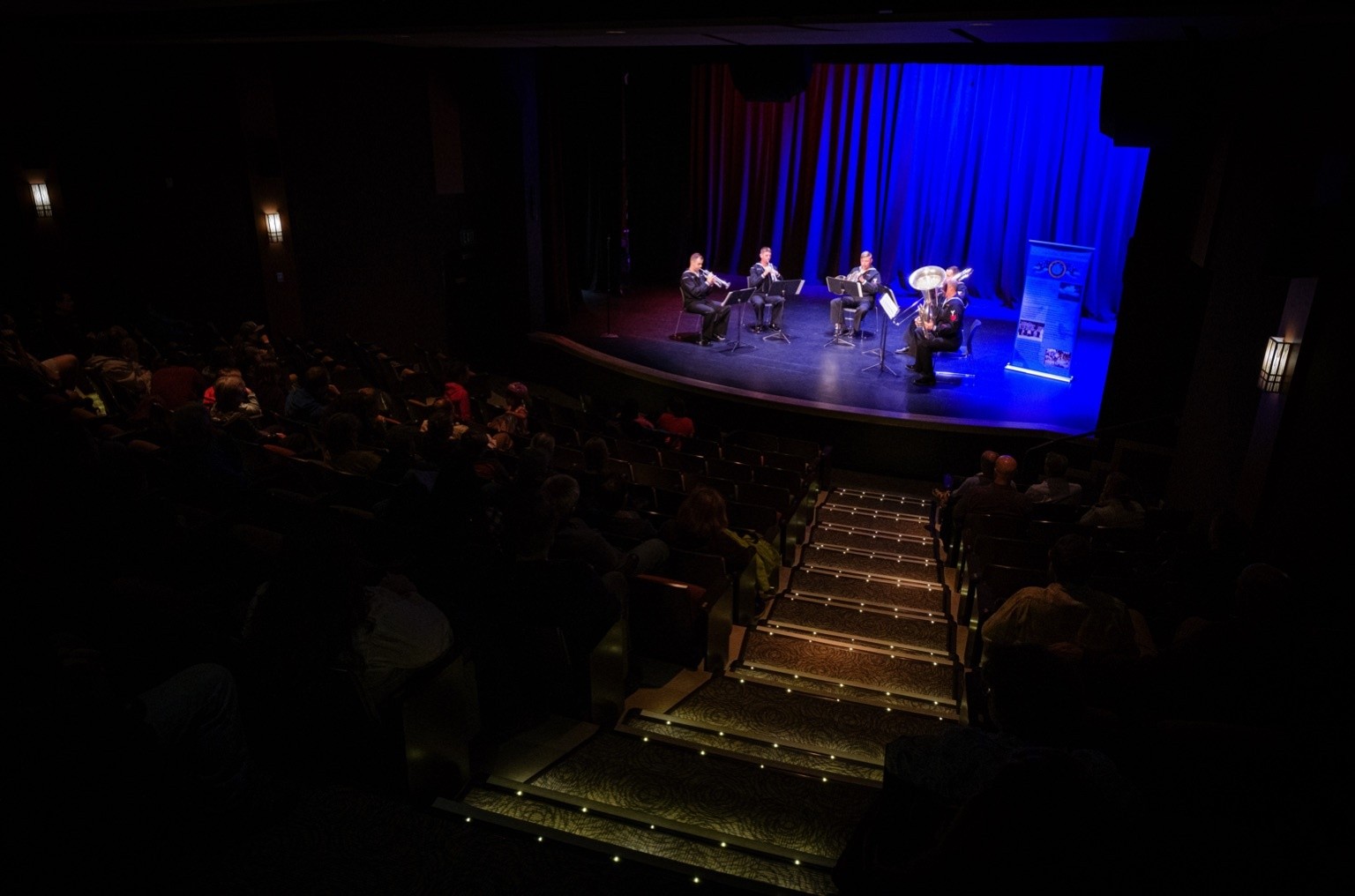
xmin=740 ymin=276 xmax=774 ymax=326
xmin=674 ymin=287 xmax=704 ymax=339
xmin=1 ymin=326 xmax=835 ymax=801
xmin=945 ymin=504 xmax=1185 ymax=685
xmin=931 ymin=318 xmax=981 ymax=371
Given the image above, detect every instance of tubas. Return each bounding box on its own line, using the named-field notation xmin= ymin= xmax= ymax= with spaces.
xmin=909 ymin=265 xmax=947 ymax=341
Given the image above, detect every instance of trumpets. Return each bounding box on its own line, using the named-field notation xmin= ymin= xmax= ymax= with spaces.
xmin=699 ymin=268 xmax=731 ymax=290
xmin=767 ymin=260 xmax=780 ymax=282
xmin=847 ymin=265 xmax=865 ymax=282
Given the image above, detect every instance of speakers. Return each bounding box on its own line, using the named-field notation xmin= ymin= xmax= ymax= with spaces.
xmin=1099 ymin=40 xmax=1162 ymax=149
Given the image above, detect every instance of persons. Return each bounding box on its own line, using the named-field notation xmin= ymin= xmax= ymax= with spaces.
xmin=830 ymin=252 xmax=881 ymax=338
xmin=750 ymin=247 xmax=784 ymax=333
xmin=1 ymin=290 xmax=1355 ymax=896
xmin=681 ymin=253 xmax=732 ymax=348
xmin=895 ymin=266 xmax=969 ymax=385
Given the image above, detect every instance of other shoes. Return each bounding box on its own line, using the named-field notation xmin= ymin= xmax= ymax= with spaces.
xmin=769 ymin=322 xmax=782 ymax=332
xmin=848 ymin=327 xmax=862 ymax=336
xmin=696 ymin=334 xmax=713 ymax=347
xmin=903 ymin=346 xmax=910 ymax=352
xmin=755 ymin=324 xmax=763 ymax=333
xmin=914 ymin=376 xmax=936 ymax=386
xmin=833 ymin=326 xmax=848 ymax=336
xmin=905 ymin=364 xmax=916 ymax=371
xmin=713 ymin=332 xmax=727 ymax=342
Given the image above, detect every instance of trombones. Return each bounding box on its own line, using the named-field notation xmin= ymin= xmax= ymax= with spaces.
xmin=892 ymin=267 xmax=973 ymax=327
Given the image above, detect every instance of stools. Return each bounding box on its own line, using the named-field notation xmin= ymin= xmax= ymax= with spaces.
xmin=842 ymin=306 xmax=878 ymax=338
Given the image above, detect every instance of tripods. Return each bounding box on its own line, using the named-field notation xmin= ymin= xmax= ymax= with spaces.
xmin=762 ymin=279 xmax=801 ymax=344
xmin=823 ymin=277 xmax=860 ymax=347
xmin=861 ymin=285 xmax=899 ymax=378
xmin=724 ymin=288 xmax=756 ymax=352
